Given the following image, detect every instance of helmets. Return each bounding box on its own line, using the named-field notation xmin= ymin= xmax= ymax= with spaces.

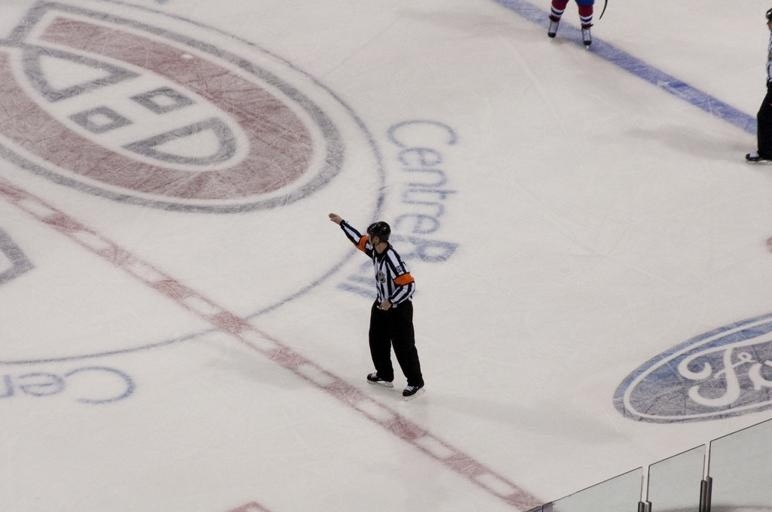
xmin=367 ymin=222 xmax=390 ymax=239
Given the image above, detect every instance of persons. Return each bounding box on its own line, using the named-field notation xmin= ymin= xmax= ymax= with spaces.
xmin=548 ymin=0 xmax=595 ymax=47
xmin=746 ymin=8 xmax=772 ymax=160
xmin=328 ymin=211 xmax=425 ymax=397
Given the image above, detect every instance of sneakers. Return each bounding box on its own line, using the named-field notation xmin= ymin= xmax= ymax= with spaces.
xmin=582 ymin=24 xmax=593 ymax=45
xmin=403 ymin=381 xmax=424 ymax=396
xmin=367 ymin=372 xmax=394 ymax=383
xmin=746 ymin=151 xmax=771 ymax=162
xmin=548 ymin=16 xmax=560 ymax=37
